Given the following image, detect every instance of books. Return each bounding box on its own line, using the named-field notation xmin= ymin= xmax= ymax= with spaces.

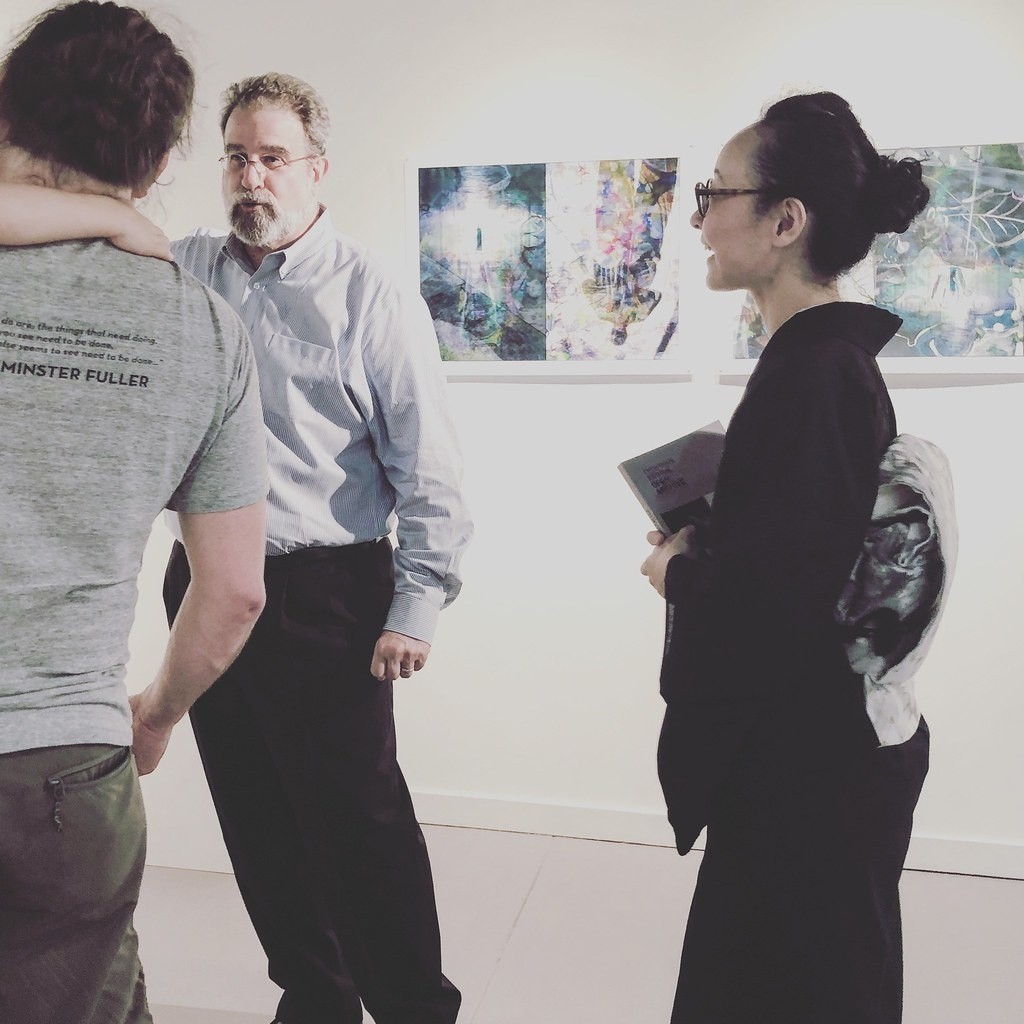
xmin=619 ymin=419 xmax=727 ymax=540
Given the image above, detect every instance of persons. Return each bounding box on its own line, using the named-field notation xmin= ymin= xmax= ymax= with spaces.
xmin=642 ymin=93 xmax=963 ymax=1024
xmin=164 ymin=70 xmax=477 ymax=1024
xmin=1 ymin=0 xmax=275 ymax=1023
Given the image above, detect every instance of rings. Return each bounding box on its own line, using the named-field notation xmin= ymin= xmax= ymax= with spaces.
xmin=400 ymin=666 xmax=415 ymax=672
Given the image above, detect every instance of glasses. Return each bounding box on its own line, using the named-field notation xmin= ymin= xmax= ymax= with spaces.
xmin=219 ymin=151 xmax=325 ymax=174
xmin=695 ymin=178 xmax=808 ymax=218
